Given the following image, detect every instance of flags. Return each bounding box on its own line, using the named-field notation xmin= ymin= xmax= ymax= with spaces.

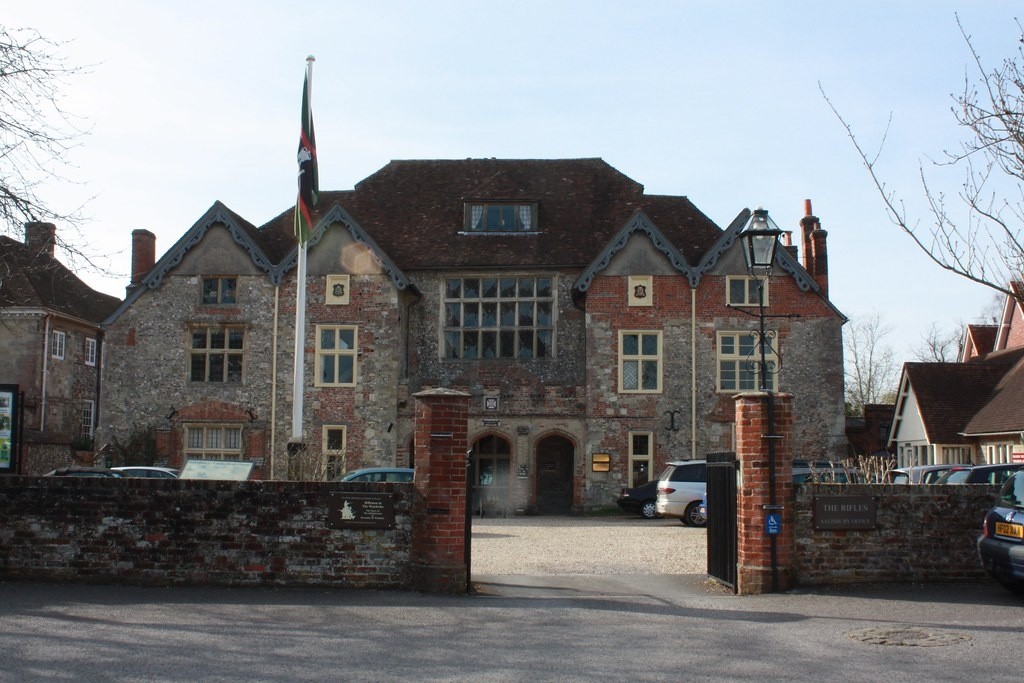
xmin=293 ymin=66 xmax=322 ymax=249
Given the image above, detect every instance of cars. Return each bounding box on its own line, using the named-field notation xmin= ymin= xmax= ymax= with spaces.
xmin=339 ymin=466 xmax=413 ymax=482
xmin=46 ymin=466 xmax=180 ymax=478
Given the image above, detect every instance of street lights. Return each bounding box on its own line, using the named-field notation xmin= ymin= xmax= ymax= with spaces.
xmin=725 ymin=207 xmax=803 ymax=390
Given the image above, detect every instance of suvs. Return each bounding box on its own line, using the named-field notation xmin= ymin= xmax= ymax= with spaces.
xmin=978 ymin=466 xmax=1024 ymax=591
xmin=615 ymin=460 xmax=1024 ymax=552
